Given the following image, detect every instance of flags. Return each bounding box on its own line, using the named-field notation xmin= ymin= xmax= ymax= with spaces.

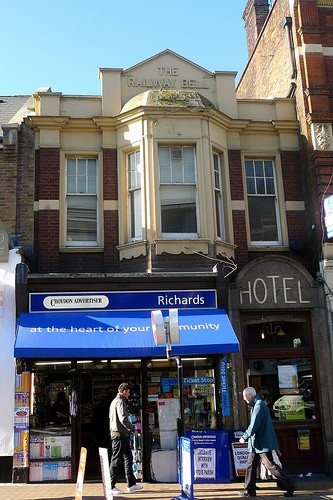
xmin=219 ymin=356 xmax=230 ymax=416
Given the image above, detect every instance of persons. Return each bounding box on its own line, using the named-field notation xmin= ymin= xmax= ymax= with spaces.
xmin=51 ymin=392 xmax=69 ymax=424
xmin=110 ymin=383 xmax=143 ymax=493
xmin=239 ymin=387 xmax=296 ymax=497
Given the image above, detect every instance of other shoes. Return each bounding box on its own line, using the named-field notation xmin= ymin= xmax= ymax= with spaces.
xmin=244 ymin=483 xmax=260 ymax=490
xmin=284 ymin=485 xmax=297 ymax=497
xmin=238 ymin=489 xmax=256 ymax=497
xmin=112 ymin=487 xmax=124 ymax=494
xmin=127 ymin=484 xmax=143 ymax=493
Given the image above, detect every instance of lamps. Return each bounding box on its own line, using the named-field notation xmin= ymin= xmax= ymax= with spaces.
xmin=260 ymin=321 xmax=285 ymax=339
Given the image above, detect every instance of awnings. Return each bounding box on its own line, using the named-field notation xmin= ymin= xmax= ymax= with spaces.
xmin=14 ymin=309 xmax=239 ymax=358
